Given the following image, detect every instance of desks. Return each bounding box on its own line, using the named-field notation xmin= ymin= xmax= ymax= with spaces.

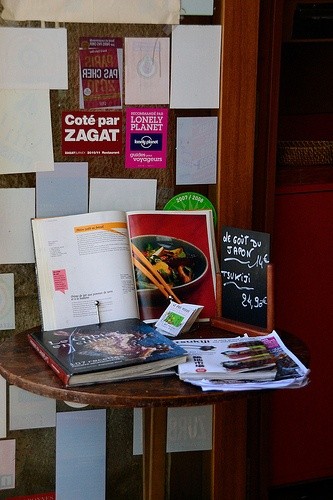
xmin=0 ymin=322 xmax=308 ymax=500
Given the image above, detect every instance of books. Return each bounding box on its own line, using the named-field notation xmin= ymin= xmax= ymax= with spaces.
xmin=171 ymin=336 xmax=278 ymax=381
xmin=31 ymin=209 xmax=221 ymax=332
xmin=27 ymin=317 xmax=189 ymax=387
